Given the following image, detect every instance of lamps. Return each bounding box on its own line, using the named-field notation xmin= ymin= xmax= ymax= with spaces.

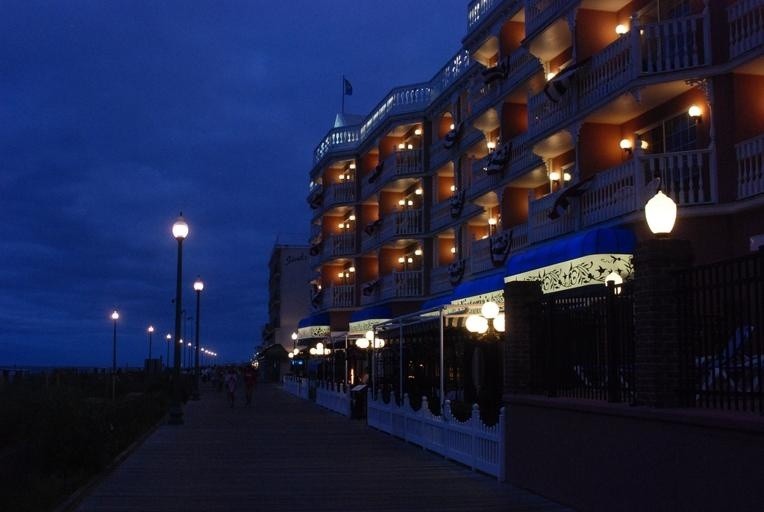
xmin=465 ymin=300 xmax=505 ymax=333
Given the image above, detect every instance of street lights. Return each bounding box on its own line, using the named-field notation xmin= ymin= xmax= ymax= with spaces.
xmin=288 ymin=332 xmax=303 ymax=382
xmin=166 ymin=333 xmax=172 ymax=370
xmin=178 ymin=338 xmax=218 ymax=367
xmin=147 ymin=325 xmax=156 ymax=361
xmin=109 ymin=310 xmax=120 ymax=404
xmin=193 ymin=275 xmax=205 ymax=372
xmin=466 ymin=302 xmax=507 ymax=358
xmin=309 ymin=342 xmax=331 ymax=359
xmin=356 ymin=331 xmax=386 ymax=389
xmin=602 ymin=267 xmax=628 ymax=299
xmin=170 ymin=206 xmax=190 ymax=378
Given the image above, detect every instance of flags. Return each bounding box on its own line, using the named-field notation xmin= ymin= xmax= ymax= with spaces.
xmin=343 ymin=79 xmax=353 ymax=96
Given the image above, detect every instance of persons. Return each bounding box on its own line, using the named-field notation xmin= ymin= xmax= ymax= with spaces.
xmin=216 ymin=367 xmax=225 ymax=392
xmin=224 ymin=368 xmax=238 ymax=408
xmin=241 ymin=364 xmax=256 ymax=407
